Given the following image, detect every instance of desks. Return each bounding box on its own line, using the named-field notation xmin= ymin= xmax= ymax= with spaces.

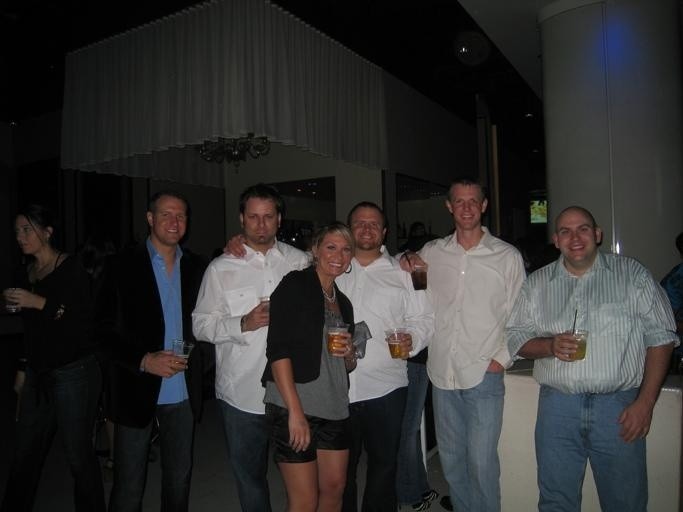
xmin=496 ymin=359 xmax=682 ymax=512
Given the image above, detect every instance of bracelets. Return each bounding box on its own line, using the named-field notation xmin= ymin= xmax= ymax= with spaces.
xmin=143 ymin=356 xmax=148 ymax=372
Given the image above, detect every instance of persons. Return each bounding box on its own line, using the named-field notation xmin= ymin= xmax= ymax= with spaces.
xmin=260 ymin=220 xmax=358 ymax=512
xmin=394 ymin=175 xmax=528 ymax=511
xmin=394 ymin=347 xmax=439 ymax=512
xmin=504 ymin=205 xmax=679 ymax=512
xmin=0 ymin=198 xmax=104 ymax=512
xmin=86 ymin=188 xmax=247 ymax=512
xmin=334 ymin=201 xmax=436 ymax=512
xmin=191 ymin=187 xmax=311 ymax=512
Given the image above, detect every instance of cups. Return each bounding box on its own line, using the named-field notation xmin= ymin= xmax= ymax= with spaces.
xmin=385 ymin=328 xmax=407 ymax=359
xmin=328 ymin=326 xmax=348 ymax=354
xmin=565 ymin=330 xmax=589 ymax=360
xmin=261 ymin=296 xmax=271 ymax=307
xmin=7 ymin=300 xmax=17 ymax=313
xmin=172 ymin=340 xmax=195 ymax=361
xmin=411 ymin=265 xmax=427 ymax=290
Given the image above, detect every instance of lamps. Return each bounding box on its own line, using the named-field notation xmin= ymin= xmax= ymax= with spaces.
xmin=200 ymin=133 xmax=271 ymax=174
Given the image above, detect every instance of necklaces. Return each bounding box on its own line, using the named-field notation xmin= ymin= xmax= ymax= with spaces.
xmin=347 ymin=352 xmax=356 ymax=365
xmin=321 ymin=284 xmax=337 ymax=304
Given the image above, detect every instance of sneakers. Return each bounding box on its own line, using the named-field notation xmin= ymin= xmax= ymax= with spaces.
xmin=412 ymin=490 xmax=452 ymax=512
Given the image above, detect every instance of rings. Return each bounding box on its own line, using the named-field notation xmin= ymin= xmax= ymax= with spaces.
xmin=11 ymin=286 xmax=17 ymax=292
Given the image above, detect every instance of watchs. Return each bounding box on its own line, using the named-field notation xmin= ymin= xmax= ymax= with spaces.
xmin=241 ymin=314 xmax=247 ymax=332
